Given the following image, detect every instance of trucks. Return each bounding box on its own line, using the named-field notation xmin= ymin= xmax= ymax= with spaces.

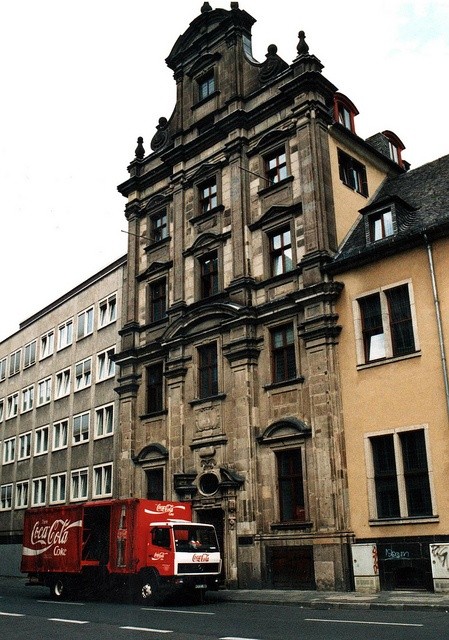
xmin=20 ymin=498 xmax=223 ymax=605
